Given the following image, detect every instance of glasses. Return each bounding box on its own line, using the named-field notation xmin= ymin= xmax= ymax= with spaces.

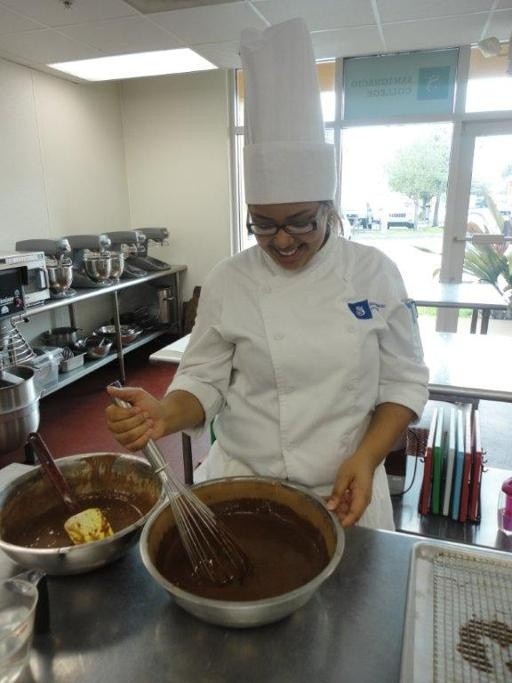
xmin=245 ymin=207 xmax=321 ymax=236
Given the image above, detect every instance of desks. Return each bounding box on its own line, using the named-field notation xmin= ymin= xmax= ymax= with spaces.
xmin=407 ymin=281 xmax=508 ymax=333
xmin=150 ymin=328 xmax=512 ymax=553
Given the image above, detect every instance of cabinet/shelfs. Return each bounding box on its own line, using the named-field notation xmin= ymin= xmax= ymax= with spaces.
xmin=6 ymin=266 xmax=188 ymax=400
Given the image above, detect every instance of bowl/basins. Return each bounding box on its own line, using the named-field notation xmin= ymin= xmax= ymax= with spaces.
xmin=1 ymin=452 xmax=167 ymax=577
xmin=139 ymin=475 xmax=347 ymax=629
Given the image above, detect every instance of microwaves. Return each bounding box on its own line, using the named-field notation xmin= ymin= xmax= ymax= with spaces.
xmin=0 ymin=251 xmax=51 ymax=317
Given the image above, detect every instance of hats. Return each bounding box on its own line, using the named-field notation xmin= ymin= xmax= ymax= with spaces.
xmin=235 ymin=17 xmax=339 ymax=206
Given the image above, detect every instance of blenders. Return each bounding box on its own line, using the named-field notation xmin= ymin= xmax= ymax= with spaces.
xmin=14 ymin=224 xmax=175 ymax=299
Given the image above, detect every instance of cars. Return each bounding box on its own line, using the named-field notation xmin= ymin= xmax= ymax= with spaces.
xmin=343 ymin=202 xmax=414 ymax=229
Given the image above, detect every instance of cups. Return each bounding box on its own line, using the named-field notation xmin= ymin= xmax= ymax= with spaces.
xmin=0 ymin=569 xmax=46 ymax=683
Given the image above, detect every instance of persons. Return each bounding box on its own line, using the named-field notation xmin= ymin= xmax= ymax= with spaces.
xmin=107 ymin=206 xmax=430 ymax=535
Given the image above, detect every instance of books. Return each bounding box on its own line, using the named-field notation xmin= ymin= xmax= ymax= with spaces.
xmin=416 ymin=405 xmax=482 ymax=523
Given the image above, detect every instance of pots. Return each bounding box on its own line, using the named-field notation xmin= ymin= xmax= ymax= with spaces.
xmin=0 ymin=362 xmax=43 ymax=454
xmin=39 ymin=323 xmax=143 ymax=360
